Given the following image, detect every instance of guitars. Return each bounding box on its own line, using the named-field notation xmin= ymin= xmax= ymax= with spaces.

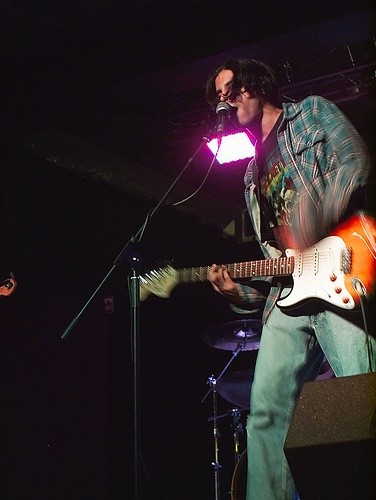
xmin=0 ymin=272 xmax=17 ymax=297
xmin=138 ymin=214 xmax=376 ymax=312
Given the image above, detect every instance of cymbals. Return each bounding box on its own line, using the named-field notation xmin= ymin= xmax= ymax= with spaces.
xmin=202 ymin=319 xmax=262 ymax=352
xmin=212 ymin=370 xmax=255 ymax=409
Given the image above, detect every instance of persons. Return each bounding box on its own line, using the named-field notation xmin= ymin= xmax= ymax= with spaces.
xmin=205 ymin=61 xmax=376 ymax=500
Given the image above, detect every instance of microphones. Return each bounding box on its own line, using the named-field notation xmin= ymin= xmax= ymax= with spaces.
xmin=215 ymin=102 xmax=231 ymax=147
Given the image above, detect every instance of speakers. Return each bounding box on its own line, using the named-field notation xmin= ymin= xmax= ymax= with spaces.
xmin=284 ymin=372 xmax=376 ymax=500
